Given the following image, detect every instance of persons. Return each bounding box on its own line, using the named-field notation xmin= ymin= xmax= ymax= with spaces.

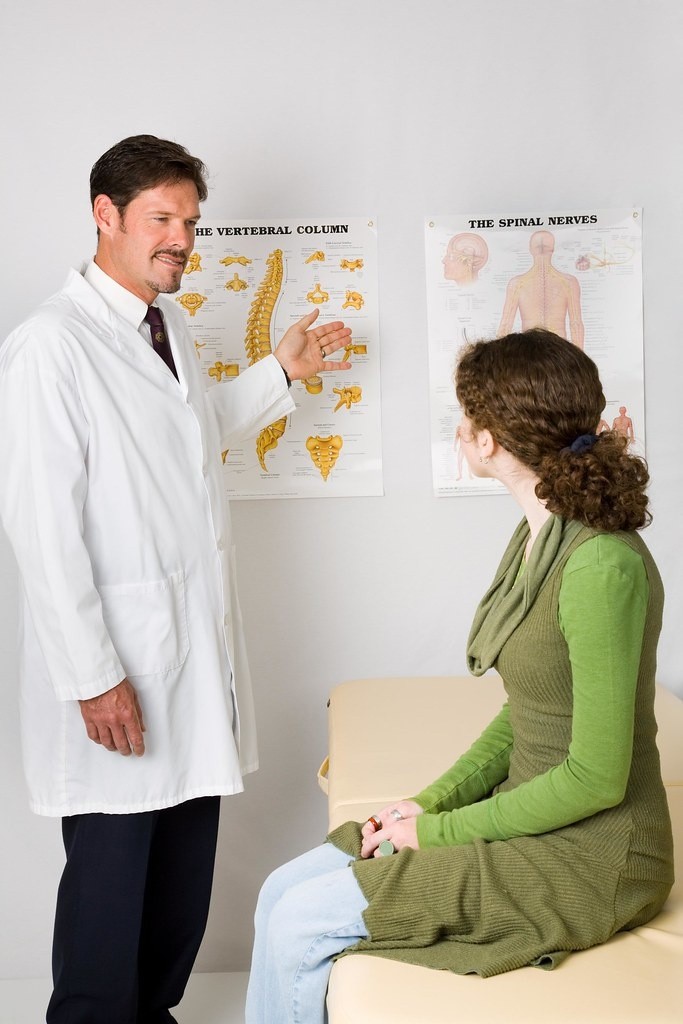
xmin=245 ymin=328 xmax=675 ymax=1024
xmin=0 ymin=135 xmax=352 ymax=1024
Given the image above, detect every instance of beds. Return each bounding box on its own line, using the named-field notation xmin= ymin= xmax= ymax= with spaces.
xmin=317 ymin=677 xmax=683 ymax=1024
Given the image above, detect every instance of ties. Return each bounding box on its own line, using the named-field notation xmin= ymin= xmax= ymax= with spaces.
xmin=144 ymin=307 xmax=179 ymax=385
